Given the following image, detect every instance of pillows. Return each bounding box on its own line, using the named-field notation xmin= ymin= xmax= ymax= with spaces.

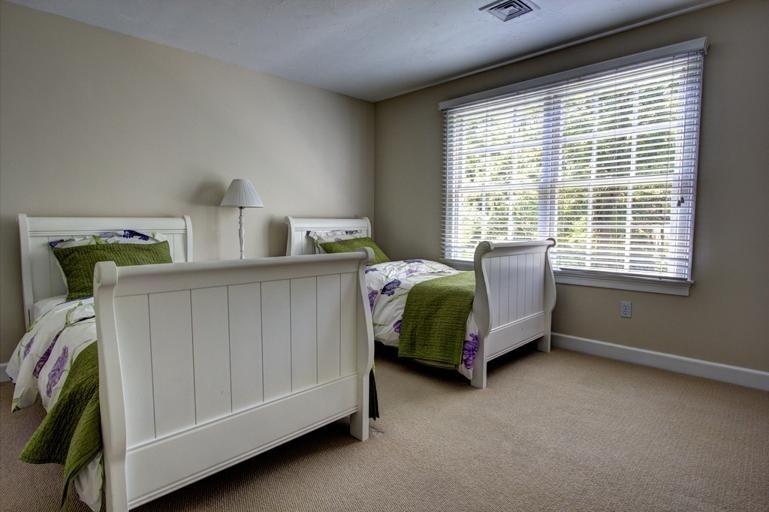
xmin=48 ymin=230 xmax=167 ymax=296
xmin=319 ymin=237 xmax=391 ymax=266
xmin=51 ymin=241 xmax=172 ymax=302
xmin=307 ymin=230 xmax=365 ymax=254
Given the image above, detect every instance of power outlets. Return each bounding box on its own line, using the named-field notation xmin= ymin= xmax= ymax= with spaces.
xmin=620 ymin=301 xmax=632 ymax=318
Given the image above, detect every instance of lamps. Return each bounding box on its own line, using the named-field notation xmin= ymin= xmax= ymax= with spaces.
xmin=219 ymin=178 xmax=264 ymax=258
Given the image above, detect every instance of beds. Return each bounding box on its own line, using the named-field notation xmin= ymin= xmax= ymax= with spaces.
xmin=18 ymin=213 xmax=375 ymax=512
xmin=283 ymin=216 xmax=557 ymax=389
